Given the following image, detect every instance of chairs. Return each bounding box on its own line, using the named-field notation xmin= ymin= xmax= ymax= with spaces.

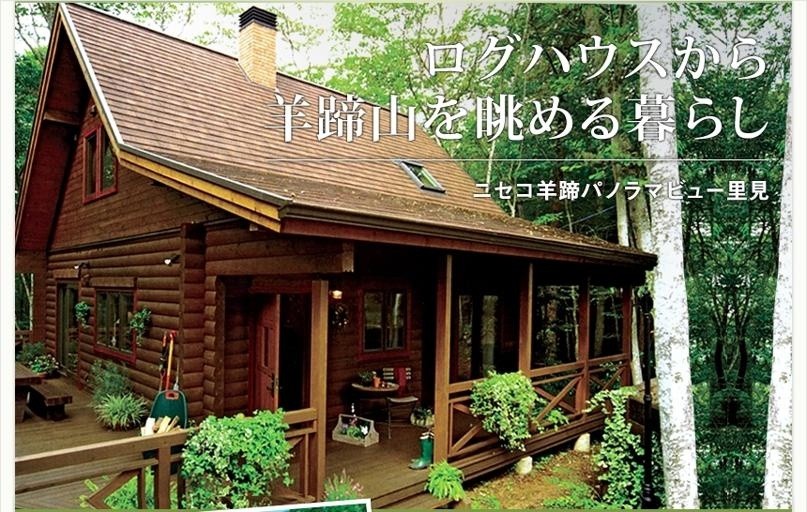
xmin=381 ymin=365 xmax=418 ymax=441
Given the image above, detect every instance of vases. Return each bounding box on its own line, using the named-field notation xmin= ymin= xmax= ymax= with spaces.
xmin=44 ymin=370 xmax=58 ymax=378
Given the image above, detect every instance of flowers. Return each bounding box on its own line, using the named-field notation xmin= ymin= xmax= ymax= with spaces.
xmin=28 ymin=353 xmax=60 ymax=373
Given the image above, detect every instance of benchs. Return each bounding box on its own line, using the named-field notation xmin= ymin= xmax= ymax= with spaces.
xmin=30 ymin=380 xmax=74 ymax=422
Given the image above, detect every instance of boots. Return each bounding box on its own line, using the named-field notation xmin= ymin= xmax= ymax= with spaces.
xmin=410 ymin=431 xmax=430 ymax=462
xmin=408 ymin=436 xmax=434 ymax=469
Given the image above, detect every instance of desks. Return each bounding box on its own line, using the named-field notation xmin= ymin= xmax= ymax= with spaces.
xmin=349 ymin=381 xmax=400 ymax=441
xmin=16 ymin=358 xmax=44 ymax=422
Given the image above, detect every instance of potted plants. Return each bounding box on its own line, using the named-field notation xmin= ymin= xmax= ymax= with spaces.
xmin=94 ymin=391 xmax=153 ymax=434
